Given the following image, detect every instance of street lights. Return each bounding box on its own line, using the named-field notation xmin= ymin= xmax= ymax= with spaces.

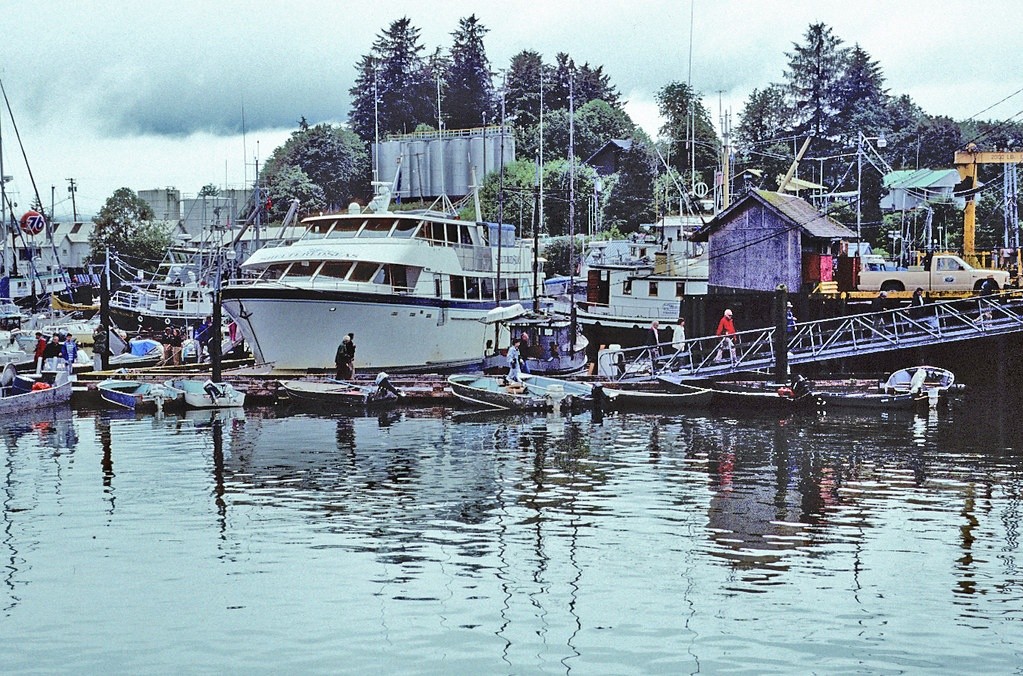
xmin=857 ymin=129 xmax=887 ymax=257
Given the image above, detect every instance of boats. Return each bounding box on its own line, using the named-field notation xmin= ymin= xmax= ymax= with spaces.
xmin=655 ymin=374 xmax=807 ymax=410
xmin=579 ymin=381 xmax=713 ymax=408
xmin=885 ymin=365 xmax=955 ymax=397
xmin=0 ymin=71 xmax=710 ymax=368
xmin=95 ymin=377 xmax=246 ymax=410
xmin=447 ymin=374 xmax=593 ymax=410
xmin=819 ymin=393 xmax=930 ymax=411
xmin=0 ymin=363 xmax=73 ymax=415
xmin=277 ymin=380 xmax=373 ymax=407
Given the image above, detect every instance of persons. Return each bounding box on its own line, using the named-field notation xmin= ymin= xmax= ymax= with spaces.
xmin=549 ymin=341 xmax=560 ymax=358
xmin=669 ymin=317 xmax=687 ymax=372
xmin=786 ymin=301 xmax=797 ymax=351
xmin=973 ymin=276 xmax=997 ymax=327
xmin=647 ymin=320 xmax=662 ymax=375
xmin=713 ymin=309 xmax=741 ymax=364
xmin=336 ymin=333 xmax=356 ymax=380
xmin=912 ymin=287 xmax=926 ymax=333
xmin=871 ymin=291 xmax=889 ymax=343
xmin=122 ymin=316 xmax=235 ymax=365
xmin=34 ymin=332 xmax=79 ymax=375
xmin=505 ymin=332 xmax=532 ymax=383
xmin=587 ymin=321 xmax=606 ymax=375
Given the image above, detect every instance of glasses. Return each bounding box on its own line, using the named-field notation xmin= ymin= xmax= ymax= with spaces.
xmin=727 ymin=314 xmax=733 ymax=317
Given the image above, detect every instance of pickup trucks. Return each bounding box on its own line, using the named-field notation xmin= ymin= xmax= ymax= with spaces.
xmin=855 ymin=256 xmax=1011 ymax=293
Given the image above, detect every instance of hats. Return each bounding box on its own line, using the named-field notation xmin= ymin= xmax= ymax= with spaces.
xmin=65 ymin=333 xmax=73 ymax=337
xmin=787 ymin=301 xmax=794 ymax=307
xmin=986 ymin=275 xmax=996 ymax=280
